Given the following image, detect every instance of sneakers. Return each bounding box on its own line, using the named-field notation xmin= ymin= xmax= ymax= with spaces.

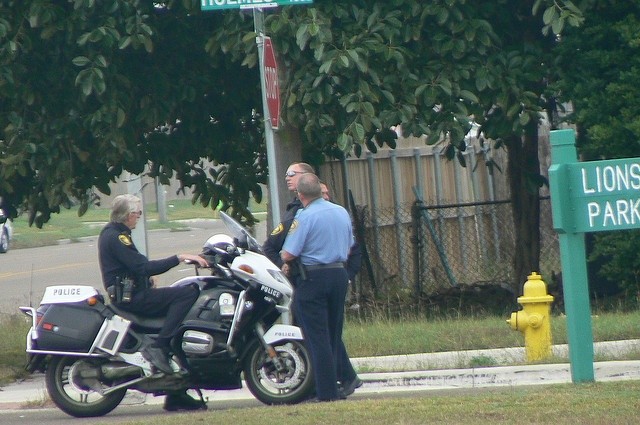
xmin=339 ymin=375 xmax=364 ymax=396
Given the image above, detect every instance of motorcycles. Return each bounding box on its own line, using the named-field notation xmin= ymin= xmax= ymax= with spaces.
xmin=17 ymin=208 xmax=318 ymax=419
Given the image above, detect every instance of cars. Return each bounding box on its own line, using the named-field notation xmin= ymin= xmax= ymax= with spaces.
xmin=0 ymin=207 xmax=12 ymax=256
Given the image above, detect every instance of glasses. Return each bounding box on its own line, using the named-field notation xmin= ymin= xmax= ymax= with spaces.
xmin=286 ymin=171 xmax=306 ymax=177
xmin=131 ymin=211 xmax=142 ymax=215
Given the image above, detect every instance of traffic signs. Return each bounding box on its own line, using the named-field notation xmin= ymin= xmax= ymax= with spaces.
xmin=201 ymin=0 xmax=313 ymax=11
xmin=565 ymin=158 xmax=640 ymax=232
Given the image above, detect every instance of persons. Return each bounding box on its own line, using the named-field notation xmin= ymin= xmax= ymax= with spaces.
xmin=263 ymin=181 xmax=362 ymax=289
xmin=284 ymin=163 xmax=315 ymax=216
xmin=280 ymin=173 xmax=362 ymax=403
xmin=98 ymin=195 xmax=209 ymax=411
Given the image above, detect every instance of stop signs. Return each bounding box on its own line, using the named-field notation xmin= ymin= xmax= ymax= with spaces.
xmin=261 ymin=36 xmax=282 ymax=132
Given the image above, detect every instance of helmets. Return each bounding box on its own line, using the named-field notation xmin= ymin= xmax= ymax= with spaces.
xmin=202 ymin=234 xmax=235 ymax=257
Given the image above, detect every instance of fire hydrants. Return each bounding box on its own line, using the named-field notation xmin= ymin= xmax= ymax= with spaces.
xmin=502 ymin=269 xmax=556 ymax=367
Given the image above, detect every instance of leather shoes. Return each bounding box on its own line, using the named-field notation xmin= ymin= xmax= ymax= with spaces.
xmin=140 ymin=343 xmax=174 ymax=375
xmin=165 ymin=394 xmax=202 ymax=411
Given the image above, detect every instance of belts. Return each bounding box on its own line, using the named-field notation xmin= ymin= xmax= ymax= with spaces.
xmin=305 ymin=262 xmax=344 ymax=272
